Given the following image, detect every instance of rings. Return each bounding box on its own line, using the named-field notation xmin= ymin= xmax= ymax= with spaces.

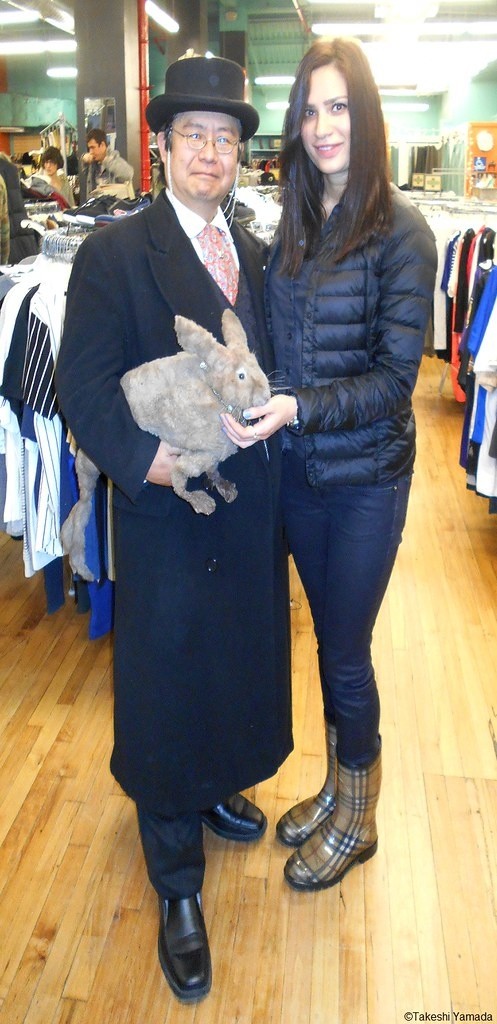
xmin=251 ymin=425 xmax=260 ymax=441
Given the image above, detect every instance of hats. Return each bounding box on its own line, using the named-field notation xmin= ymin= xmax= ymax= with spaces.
xmin=146 ymin=56 xmax=260 ymax=145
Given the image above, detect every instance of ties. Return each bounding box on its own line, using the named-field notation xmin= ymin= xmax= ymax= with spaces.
xmin=195 ymin=224 xmax=240 ymax=307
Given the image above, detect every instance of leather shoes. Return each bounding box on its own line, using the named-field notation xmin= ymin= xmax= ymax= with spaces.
xmin=200 ymin=792 xmax=268 ymax=843
xmin=156 ymin=889 xmax=213 ymax=1003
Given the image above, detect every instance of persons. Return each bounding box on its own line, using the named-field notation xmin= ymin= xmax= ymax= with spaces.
xmin=219 ymin=36 xmax=439 ymax=892
xmin=36 ymin=145 xmax=76 ymax=210
xmin=78 ymin=127 xmax=134 ymax=202
xmin=47 ymin=57 xmax=294 ymax=1004
xmin=11 ymin=151 xmax=36 ymax=180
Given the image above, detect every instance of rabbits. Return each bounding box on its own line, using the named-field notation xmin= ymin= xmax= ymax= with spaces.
xmin=62 ymin=307 xmax=286 ymax=582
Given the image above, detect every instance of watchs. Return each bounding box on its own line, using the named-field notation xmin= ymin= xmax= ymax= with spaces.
xmin=287 ymin=416 xmax=300 ymax=432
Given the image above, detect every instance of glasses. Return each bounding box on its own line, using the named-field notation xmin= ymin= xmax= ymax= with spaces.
xmin=170 ymin=128 xmax=242 ymax=155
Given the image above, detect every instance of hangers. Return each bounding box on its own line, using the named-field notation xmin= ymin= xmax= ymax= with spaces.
xmin=389 ymin=138 xmax=438 ymax=148
xmin=26 ymin=200 xmax=61 ymax=222
xmin=31 ymin=222 xmax=278 ymax=280
xmin=402 ymin=191 xmax=497 ymax=233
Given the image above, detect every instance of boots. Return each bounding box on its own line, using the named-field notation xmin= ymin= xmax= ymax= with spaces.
xmin=284 ymin=735 xmax=384 ymax=893
xmin=275 ymin=710 xmax=339 ymax=847
